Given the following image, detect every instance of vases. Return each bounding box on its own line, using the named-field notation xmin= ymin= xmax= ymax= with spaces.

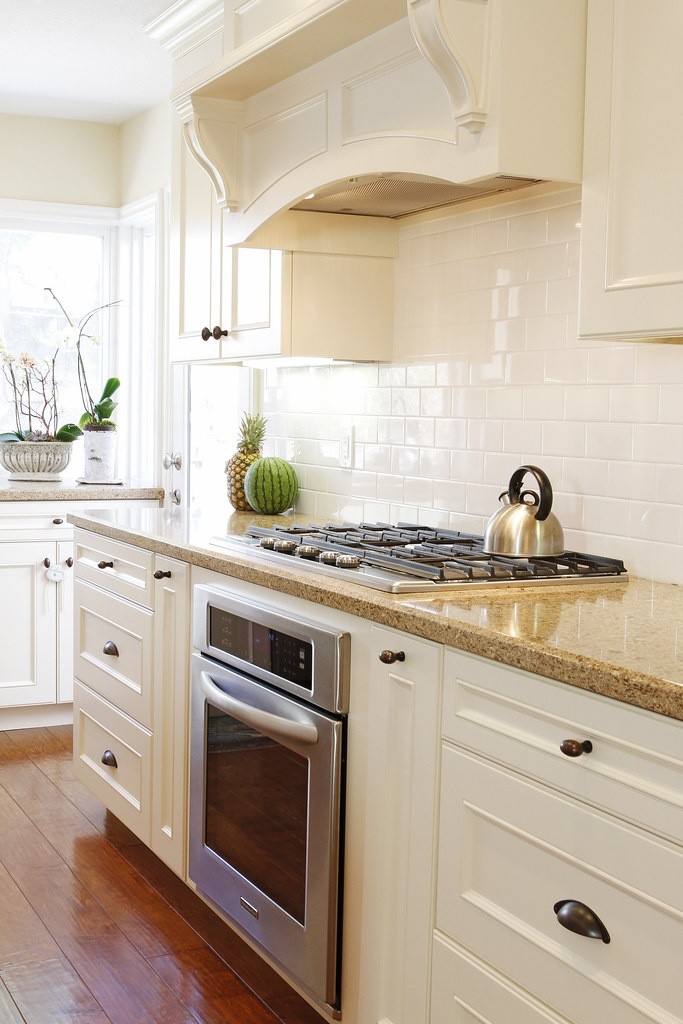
xmin=0 ymin=442 xmax=71 ymax=482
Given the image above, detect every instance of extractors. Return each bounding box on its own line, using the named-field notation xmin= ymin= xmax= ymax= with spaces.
xmin=289 ymin=176 xmax=552 ymax=220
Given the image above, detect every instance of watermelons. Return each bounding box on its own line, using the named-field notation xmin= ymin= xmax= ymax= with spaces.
xmin=244 ymin=457 xmax=298 ymax=515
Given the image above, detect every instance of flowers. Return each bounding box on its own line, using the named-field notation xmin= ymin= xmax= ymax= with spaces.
xmin=0 ymin=325 xmax=85 ymax=442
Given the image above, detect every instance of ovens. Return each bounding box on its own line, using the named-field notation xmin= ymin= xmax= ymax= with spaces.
xmin=188 ymin=584 xmax=351 ymax=1023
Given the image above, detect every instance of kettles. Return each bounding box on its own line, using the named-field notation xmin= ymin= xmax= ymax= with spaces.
xmin=481 ymin=466 xmax=564 ymax=557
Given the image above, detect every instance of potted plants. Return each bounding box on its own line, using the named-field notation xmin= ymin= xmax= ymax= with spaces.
xmin=42 ymin=287 xmax=125 ymax=484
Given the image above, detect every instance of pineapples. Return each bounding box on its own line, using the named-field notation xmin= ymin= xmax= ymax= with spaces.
xmin=227 ymin=411 xmax=269 ymax=510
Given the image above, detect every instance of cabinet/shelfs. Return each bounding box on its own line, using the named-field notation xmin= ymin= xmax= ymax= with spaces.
xmin=73 ymin=527 xmax=155 ymax=883
xmin=578 ymin=0 xmax=683 ymax=342
xmin=155 ymin=554 xmax=190 ymax=883
xmin=354 ymin=626 xmax=683 ymax=1024
xmin=0 ymin=500 xmax=160 ymax=708
xmin=144 ymin=1 xmax=585 ymax=249
xmin=143 ymin=0 xmax=394 ymax=364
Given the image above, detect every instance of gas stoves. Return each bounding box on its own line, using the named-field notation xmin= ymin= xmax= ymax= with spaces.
xmin=208 ymin=522 xmax=630 ymax=593
